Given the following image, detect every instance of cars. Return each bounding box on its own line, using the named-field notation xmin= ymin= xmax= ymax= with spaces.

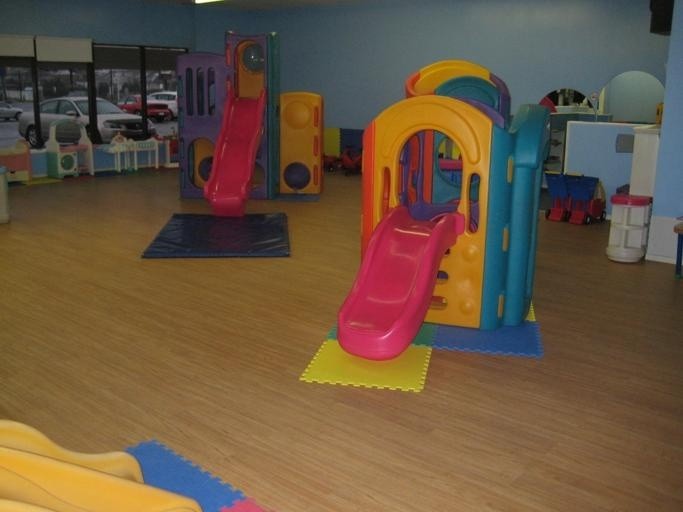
xmin=115 ymin=89 xmax=179 ymax=122
xmin=0 ymin=101 xmax=23 ymax=122
xmin=17 ymin=94 xmax=156 ymax=145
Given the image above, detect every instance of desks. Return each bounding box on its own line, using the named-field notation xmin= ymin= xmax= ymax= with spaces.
xmin=629 ymin=125 xmax=659 ymax=196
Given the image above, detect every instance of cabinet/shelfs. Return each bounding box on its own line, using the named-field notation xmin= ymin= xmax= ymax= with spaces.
xmin=540 ymin=114 xmax=612 ymax=189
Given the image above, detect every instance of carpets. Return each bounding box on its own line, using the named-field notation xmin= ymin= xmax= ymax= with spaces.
xmin=144 ymin=213 xmax=290 ymax=258
